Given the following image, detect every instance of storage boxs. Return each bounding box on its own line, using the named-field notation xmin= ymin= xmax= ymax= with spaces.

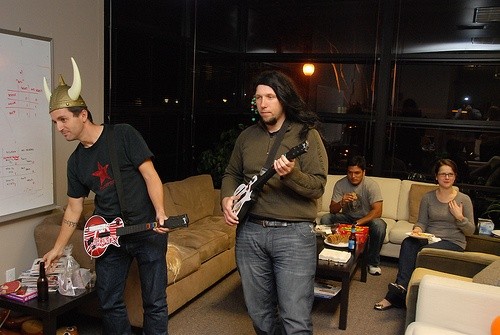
xmin=336 ymin=224 xmax=369 ymax=243
xmin=478 ymin=218 xmax=494 ymax=234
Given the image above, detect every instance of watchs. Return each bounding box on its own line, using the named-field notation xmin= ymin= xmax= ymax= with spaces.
xmin=354 ymin=221 xmax=358 ymax=226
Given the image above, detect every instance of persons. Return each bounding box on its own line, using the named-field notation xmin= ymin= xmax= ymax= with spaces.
xmin=219 ymin=72 xmax=328 ymax=335
xmin=320 ymin=157 xmax=387 ymax=276
xmin=41 ymin=57 xmax=171 ymax=335
xmin=374 ymin=159 xmax=476 ymax=311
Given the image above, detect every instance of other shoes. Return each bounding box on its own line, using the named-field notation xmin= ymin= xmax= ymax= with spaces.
xmin=366 ymin=264 xmax=382 ymax=276
xmin=374 ymin=300 xmax=393 ymax=311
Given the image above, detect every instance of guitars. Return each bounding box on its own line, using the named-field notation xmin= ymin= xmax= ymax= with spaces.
xmin=83 ymin=214 xmax=190 ymax=258
xmin=231 ymin=139 xmax=309 ymax=219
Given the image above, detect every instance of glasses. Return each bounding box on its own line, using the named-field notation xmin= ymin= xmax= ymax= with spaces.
xmin=437 ymin=172 xmax=455 ymax=177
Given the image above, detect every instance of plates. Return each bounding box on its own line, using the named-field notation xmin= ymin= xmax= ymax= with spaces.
xmin=407 ymin=232 xmax=433 ymax=238
xmin=324 ymin=238 xmax=349 ymax=248
xmin=492 ymin=230 xmax=500 ymax=236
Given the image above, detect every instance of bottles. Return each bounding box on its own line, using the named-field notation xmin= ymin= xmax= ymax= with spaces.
xmin=348 ymin=224 xmax=356 ymax=252
xmin=37 ymin=262 xmax=49 ymax=302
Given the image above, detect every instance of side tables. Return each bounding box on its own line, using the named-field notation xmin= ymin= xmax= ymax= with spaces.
xmin=0 ymin=278 xmax=105 ymax=335
xmin=466 ymin=228 xmax=500 ymax=256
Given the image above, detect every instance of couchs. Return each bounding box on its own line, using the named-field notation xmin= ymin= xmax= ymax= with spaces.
xmin=35 ymin=174 xmax=237 ymax=327
xmin=317 ymin=175 xmax=459 ymax=258
xmin=405 ymin=248 xmax=500 ymax=335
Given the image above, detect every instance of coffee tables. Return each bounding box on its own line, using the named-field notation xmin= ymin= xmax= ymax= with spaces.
xmin=315 ymin=224 xmax=370 ymax=330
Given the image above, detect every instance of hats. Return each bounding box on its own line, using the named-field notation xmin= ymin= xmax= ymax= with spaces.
xmin=42 ymin=56 xmax=88 ymax=114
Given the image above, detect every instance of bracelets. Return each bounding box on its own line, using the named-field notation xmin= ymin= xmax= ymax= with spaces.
xmin=339 ymin=201 xmax=344 ymax=208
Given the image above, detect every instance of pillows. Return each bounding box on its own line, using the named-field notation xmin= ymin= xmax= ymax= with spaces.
xmin=408 ymin=184 xmax=438 ymax=223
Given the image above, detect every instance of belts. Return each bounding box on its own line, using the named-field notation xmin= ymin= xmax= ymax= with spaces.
xmin=247 ymin=216 xmax=293 ymax=228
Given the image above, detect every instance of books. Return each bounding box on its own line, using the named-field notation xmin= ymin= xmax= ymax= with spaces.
xmin=19 ymin=258 xmax=66 ymax=292
